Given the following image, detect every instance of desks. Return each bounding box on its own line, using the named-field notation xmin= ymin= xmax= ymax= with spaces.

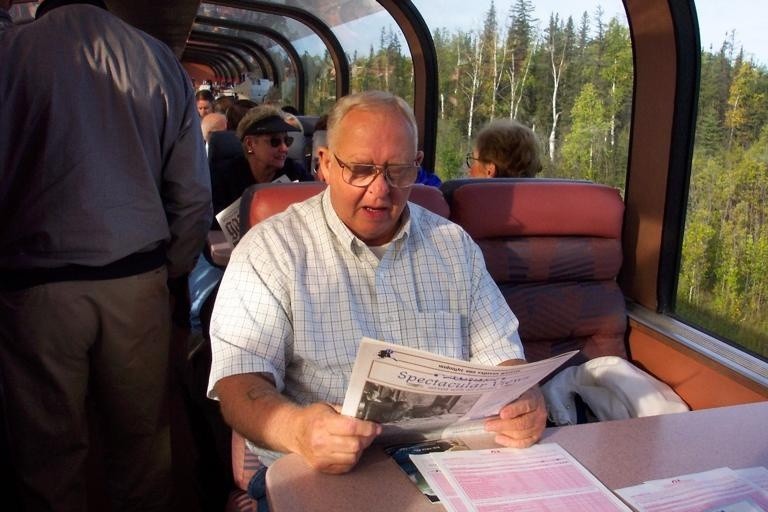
xmin=264 ymin=400 xmax=768 ymax=512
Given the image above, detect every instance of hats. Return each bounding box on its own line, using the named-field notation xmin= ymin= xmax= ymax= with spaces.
xmin=236 ymin=103 xmax=302 ymax=141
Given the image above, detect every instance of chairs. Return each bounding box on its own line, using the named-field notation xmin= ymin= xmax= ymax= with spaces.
xmin=198 ymin=116 xmax=627 ymax=512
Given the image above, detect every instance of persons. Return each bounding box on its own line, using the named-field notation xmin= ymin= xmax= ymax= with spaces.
xmin=206 ymin=91 xmax=548 ymax=511
xmin=0 ymin=0 xmax=214 ymax=512
xmin=469 ymin=118 xmax=543 ymax=179
xmin=196 ymin=91 xmax=330 ymax=230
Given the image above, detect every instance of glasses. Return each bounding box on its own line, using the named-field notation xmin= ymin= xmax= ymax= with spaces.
xmin=270 ymin=135 xmax=295 ymax=148
xmin=333 ymin=156 xmax=422 ymax=190
xmin=465 ymin=152 xmax=480 ymax=169
